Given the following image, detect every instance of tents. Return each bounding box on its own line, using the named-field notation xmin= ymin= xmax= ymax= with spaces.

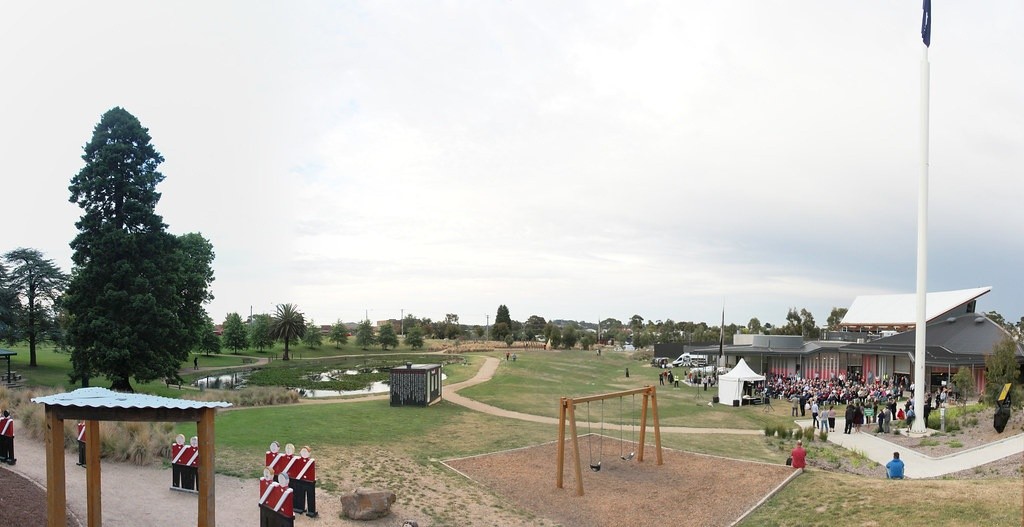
xmin=717 ymin=358 xmax=766 ymax=406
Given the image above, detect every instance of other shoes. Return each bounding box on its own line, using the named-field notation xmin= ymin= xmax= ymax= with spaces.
xmin=909 ymin=423 xmax=912 ymax=430
xmin=818 ymin=428 xmax=819 ymax=429
xmin=802 ymin=415 xmax=805 ymax=416
xmin=884 ymin=432 xmax=887 ymax=433
xmin=855 ymin=432 xmax=859 ymax=434
xmin=813 ymin=427 xmax=816 ymax=428
xmin=887 ymin=432 xmax=890 ymax=433
xmin=830 ymin=431 xmax=834 ymax=432
xmin=796 ymin=415 xmax=798 ymax=417
xmin=906 ymin=431 xmax=909 ymax=433
xmin=792 ymin=415 xmax=794 ymax=416
xmin=845 ymin=432 xmax=850 ymax=434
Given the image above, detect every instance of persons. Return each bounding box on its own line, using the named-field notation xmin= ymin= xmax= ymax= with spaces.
xmin=193 ymin=357 xmax=199 ymax=370
xmin=506 ymin=350 xmax=516 ymax=361
xmin=596 ymin=348 xmax=601 ymax=356
xmin=885 ymin=452 xmax=905 ymax=479
xmin=0 ymin=410 xmax=317 ymax=527
xmin=659 ymin=370 xmax=679 ymax=387
xmin=685 ymin=364 xmax=960 ymax=434
xmin=786 ymin=439 xmax=806 ymax=468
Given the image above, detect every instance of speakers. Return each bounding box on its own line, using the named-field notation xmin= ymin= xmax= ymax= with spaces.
xmin=763 ymin=397 xmax=769 ymax=404
xmin=733 ymin=400 xmax=739 ymax=406
xmin=713 ymin=396 xmax=719 ymax=403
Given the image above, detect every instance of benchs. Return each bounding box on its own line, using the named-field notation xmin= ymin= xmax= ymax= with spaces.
xmin=165 ymin=379 xmax=181 ymax=390
xmin=243 ymin=358 xmax=252 ymax=363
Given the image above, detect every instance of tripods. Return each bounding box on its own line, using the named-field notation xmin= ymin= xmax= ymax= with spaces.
xmin=763 ymin=399 xmax=775 ymax=413
xmin=694 ymin=380 xmax=703 ymax=399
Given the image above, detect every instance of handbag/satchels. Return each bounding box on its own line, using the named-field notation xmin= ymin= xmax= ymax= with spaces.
xmin=786 ymin=458 xmax=792 ymax=466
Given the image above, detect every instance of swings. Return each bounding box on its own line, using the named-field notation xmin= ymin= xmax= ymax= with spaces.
xmin=586 ymin=399 xmax=604 ymax=472
xmin=619 ymin=394 xmax=636 ymax=461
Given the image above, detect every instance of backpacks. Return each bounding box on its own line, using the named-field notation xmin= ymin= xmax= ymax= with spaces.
xmin=909 ymin=410 xmax=915 ymax=420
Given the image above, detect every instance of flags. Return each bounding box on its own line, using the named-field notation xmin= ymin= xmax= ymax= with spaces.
xmin=717 ymin=318 xmax=724 ymax=358
